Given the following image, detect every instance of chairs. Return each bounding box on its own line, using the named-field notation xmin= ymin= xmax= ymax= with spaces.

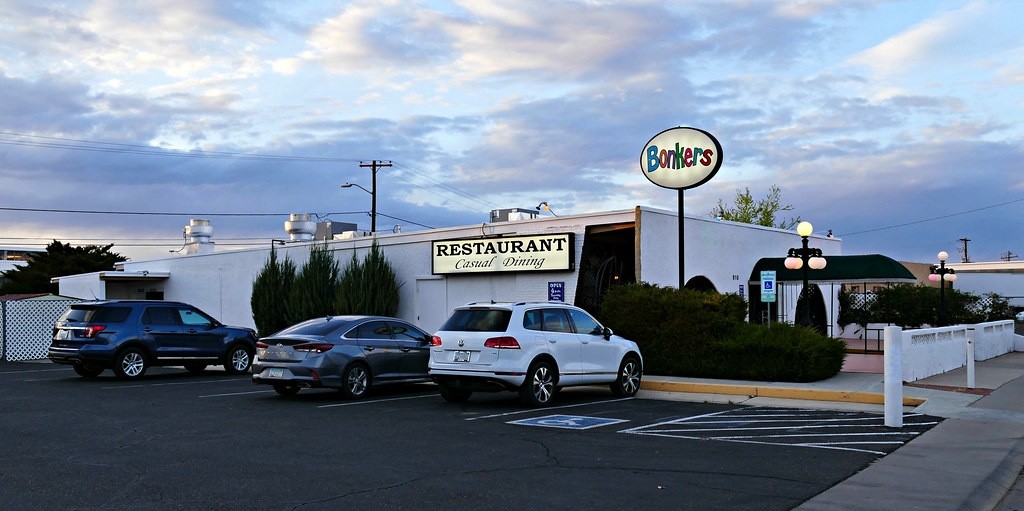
xmin=546 ymin=315 xmax=562 ymax=331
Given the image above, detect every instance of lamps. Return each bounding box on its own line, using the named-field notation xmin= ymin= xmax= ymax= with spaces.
xmin=544 ymin=202 xmax=549 ymax=210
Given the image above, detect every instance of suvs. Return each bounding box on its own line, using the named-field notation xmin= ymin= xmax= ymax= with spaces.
xmin=428 ymin=298 xmax=644 ymax=408
xmin=46 ymin=298 xmax=259 ymax=383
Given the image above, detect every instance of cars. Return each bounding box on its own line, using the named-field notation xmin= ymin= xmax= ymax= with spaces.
xmin=251 ymin=314 xmax=432 ymax=402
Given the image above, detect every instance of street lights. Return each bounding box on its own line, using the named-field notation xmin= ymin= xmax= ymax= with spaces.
xmin=927 ymin=252 xmax=957 ymax=326
xmin=784 ymin=222 xmax=828 ymax=327
xmin=340 ymin=182 xmax=376 ymax=232
